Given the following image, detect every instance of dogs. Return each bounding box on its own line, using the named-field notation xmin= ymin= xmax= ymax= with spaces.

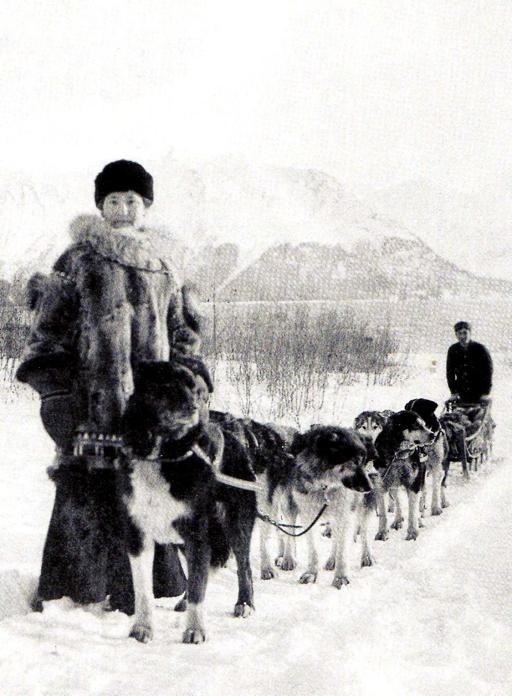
xmin=356 ymin=393 xmax=451 ymax=547
xmin=202 ymin=398 xmax=298 ymax=583
xmin=286 ymin=419 xmax=375 ymax=591
xmin=120 ymin=354 xmax=256 ymax=649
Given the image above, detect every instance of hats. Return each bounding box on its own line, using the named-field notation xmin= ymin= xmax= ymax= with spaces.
xmin=95 ymin=162 xmax=154 ymax=209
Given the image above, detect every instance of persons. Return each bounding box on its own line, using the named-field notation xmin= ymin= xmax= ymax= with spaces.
xmin=445 ymin=321 xmax=495 ymax=423
xmin=14 ymin=155 xmax=216 ymax=615
